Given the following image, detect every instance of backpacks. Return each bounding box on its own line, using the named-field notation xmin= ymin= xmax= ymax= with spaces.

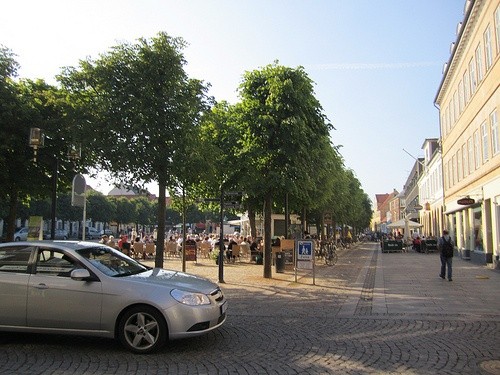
xmin=442 ymin=236 xmax=454 ymax=258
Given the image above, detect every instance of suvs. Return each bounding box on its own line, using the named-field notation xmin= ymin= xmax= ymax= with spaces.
xmin=13 ymin=226 xmax=28 ymax=242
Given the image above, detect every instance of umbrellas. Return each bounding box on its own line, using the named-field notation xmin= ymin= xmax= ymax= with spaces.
xmin=386 ymin=219 xmax=423 ymax=230
xmin=404 ymin=216 xmax=412 ymax=251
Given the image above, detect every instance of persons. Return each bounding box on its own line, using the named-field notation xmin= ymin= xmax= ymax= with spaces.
xmin=224 ymin=229 xmax=263 ymax=265
xmin=164 ymin=231 xmax=183 ymax=258
xmin=270 ymin=235 xmax=285 ymax=246
xmin=186 ymin=231 xmax=220 ymax=265
xmin=99 ymin=228 xmax=158 ymax=259
xmin=438 ymin=229 xmax=455 ymax=281
xmin=302 ymin=229 xmax=437 ymax=263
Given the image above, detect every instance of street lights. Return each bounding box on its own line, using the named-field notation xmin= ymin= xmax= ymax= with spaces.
xmin=28 ymin=117 xmax=82 ymax=257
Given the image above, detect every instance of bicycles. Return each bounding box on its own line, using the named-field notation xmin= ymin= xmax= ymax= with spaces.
xmin=323 ymin=237 xmax=337 ymax=267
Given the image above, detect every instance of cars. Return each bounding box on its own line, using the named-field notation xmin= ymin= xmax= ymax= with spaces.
xmin=45 ymin=229 xmax=68 ymax=240
xmin=0 ymin=240 xmax=227 ymax=354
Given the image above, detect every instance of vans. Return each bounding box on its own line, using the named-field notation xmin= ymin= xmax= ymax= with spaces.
xmin=78 ymin=227 xmax=102 ymax=240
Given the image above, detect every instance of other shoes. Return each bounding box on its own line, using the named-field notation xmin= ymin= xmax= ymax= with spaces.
xmin=448 ymin=279 xmax=453 ymax=281
xmin=440 ymin=274 xmax=445 ymax=279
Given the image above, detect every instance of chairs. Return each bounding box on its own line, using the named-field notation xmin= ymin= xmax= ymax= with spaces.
xmin=131 ymin=236 xmax=280 ymax=263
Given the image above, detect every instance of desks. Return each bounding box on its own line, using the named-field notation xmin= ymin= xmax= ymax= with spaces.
xmin=115 ymin=244 xmax=157 ymax=253
